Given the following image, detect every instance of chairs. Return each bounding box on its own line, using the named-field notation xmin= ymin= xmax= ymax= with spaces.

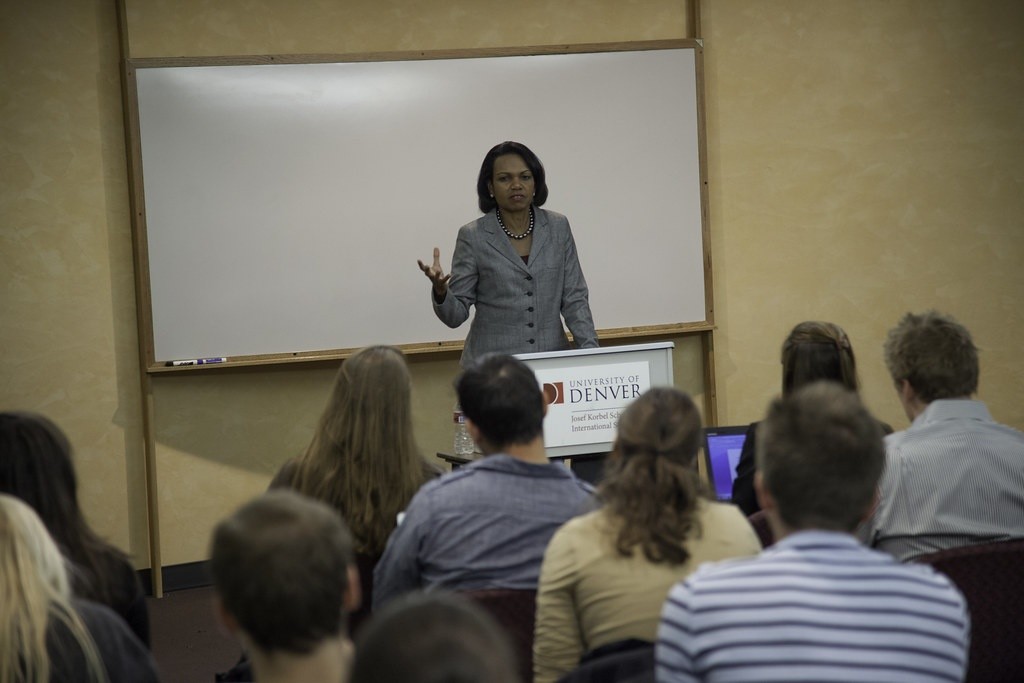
xmin=450 ymin=510 xmax=1024 ymax=682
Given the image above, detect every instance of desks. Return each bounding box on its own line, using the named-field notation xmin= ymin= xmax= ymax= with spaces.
xmin=436 ymin=442 xmax=613 ymax=471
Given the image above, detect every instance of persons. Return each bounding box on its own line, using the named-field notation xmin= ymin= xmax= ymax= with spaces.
xmin=268 ymin=347 xmax=444 ymax=640
xmin=209 ymin=490 xmax=358 ymax=683
xmin=0 ymin=409 xmax=149 ymax=650
xmin=731 ymin=321 xmax=894 ymax=552
xmin=654 ymin=380 xmax=970 ymax=683
xmin=418 ymin=141 xmax=604 ymax=486
xmin=348 ymin=597 xmax=513 ymax=682
xmin=374 ymin=352 xmax=603 ymax=619
xmin=533 ymin=387 xmax=762 ymax=683
xmin=869 ymin=308 xmax=1020 ymax=559
xmin=0 ymin=495 xmax=159 ymax=683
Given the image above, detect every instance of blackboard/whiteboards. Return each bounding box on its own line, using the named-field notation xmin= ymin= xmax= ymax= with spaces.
xmin=125 ymin=39 xmax=715 ymax=374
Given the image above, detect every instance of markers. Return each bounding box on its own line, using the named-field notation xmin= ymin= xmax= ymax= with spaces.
xmin=165 ymin=357 xmax=227 ymax=367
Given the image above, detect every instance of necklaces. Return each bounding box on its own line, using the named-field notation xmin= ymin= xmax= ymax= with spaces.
xmin=497 ymin=207 xmax=534 ymax=240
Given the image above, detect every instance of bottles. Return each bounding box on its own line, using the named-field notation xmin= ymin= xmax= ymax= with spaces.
xmin=452 ymin=400 xmax=475 ymax=454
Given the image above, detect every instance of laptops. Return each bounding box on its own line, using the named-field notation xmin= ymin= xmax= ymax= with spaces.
xmin=701 ymin=426 xmax=748 ymax=503
